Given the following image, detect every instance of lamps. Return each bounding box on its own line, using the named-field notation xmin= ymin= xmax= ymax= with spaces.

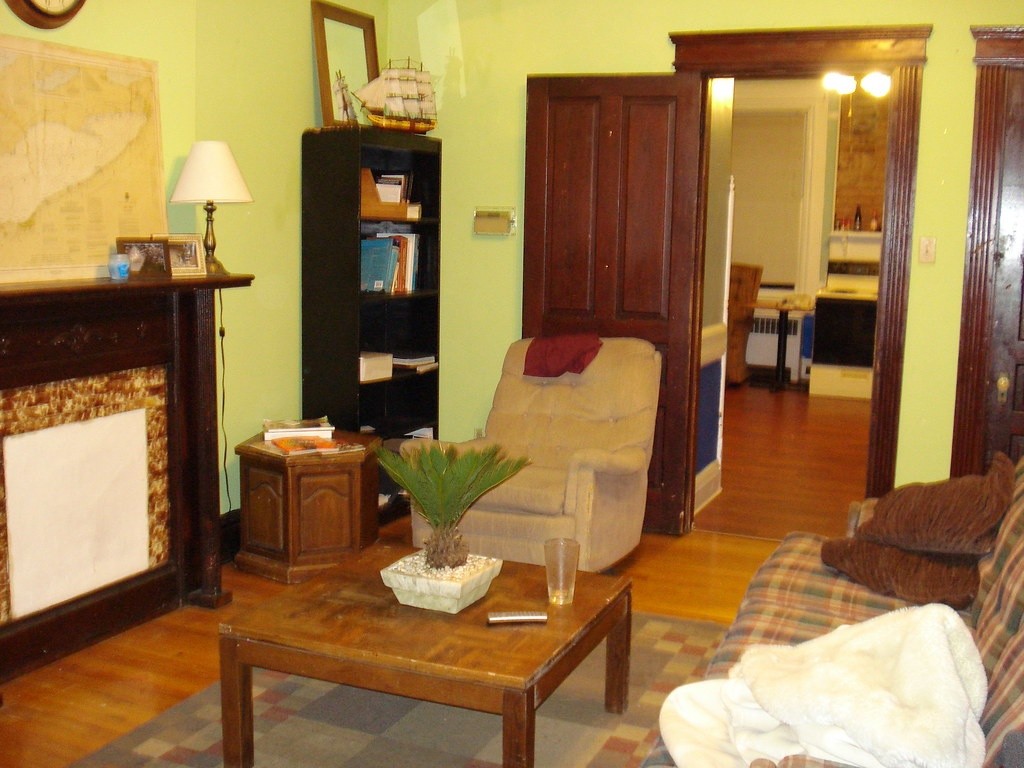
xmin=170 ymin=141 xmax=255 ymax=274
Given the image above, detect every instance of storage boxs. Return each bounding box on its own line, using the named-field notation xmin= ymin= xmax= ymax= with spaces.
xmin=358 ymin=351 xmax=394 ymax=385
xmin=360 ymin=167 xmax=408 ymax=221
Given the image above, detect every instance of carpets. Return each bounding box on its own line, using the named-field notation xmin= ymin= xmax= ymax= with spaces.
xmin=56 ymin=611 xmax=732 ymax=768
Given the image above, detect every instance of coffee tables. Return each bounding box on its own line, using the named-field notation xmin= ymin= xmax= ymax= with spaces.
xmin=738 ymin=296 xmax=816 ymax=396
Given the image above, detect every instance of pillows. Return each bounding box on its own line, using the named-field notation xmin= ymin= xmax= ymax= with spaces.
xmin=857 ymin=450 xmax=1018 ymax=557
xmin=821 ymin=536 xmax=981 ymax=611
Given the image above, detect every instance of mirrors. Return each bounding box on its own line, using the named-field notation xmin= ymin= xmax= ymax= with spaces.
xmin=310 ymin=0 xmax=382 ymax=128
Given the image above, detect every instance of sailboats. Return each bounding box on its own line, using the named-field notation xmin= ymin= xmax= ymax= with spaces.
xmin=351 ymin=56 xmax=438 ymax=133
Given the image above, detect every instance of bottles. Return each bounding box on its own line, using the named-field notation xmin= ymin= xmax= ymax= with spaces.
xmin=108 ymin=253 xmax=130 ymax=279
xmin=853 ymin=205 xmax=862 ymax=233
xmin=870 ymin=208 xmax=878 ymax=231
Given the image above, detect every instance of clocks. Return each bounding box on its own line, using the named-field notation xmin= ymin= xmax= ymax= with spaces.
xmin=5 ymin=0 xmax=87 ymax=30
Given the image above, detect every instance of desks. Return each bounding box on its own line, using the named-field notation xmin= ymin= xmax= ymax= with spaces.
xmin=212 ymin=539 xmax=631 ymax=768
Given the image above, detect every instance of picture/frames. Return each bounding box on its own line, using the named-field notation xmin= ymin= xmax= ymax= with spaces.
xmin=116 ymin=236 xmax=173 ymax=279
xmin=152 ymin=232 xmax=208 ymax=280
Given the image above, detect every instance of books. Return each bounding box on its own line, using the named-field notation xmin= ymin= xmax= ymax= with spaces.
xmin=392 ymin=355 xmax=438 ymax=374
xmin=361 ymin=233 xmax=420 ymax=294
xmin=263 ymin=416 xmax=366 ymax=456
xmin=377 ymin=168 xmax=413 ymax=204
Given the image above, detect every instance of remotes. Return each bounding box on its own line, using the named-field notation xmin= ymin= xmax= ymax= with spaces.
xmin=488 ymin=610 xmax=547 ymax=625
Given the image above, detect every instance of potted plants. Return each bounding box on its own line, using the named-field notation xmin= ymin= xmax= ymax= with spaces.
xmin=369 ymin=440 xmax=534 ymax=617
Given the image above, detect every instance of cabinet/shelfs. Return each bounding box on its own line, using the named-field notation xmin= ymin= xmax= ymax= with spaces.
xmin=301 ymin=126 xmax=442 ymax=527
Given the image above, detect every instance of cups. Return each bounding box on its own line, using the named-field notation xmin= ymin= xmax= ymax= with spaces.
xmin=544 ymin=537 xmax=580 ymax=607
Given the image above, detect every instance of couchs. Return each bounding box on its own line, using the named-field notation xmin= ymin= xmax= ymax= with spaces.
xmin=398 ymin=336 xmax=663 ymax=573
xmin=632 ymin=456 xmax=1024 ymax=768
xmin=729 ymin=261 xmax=764 ymax=386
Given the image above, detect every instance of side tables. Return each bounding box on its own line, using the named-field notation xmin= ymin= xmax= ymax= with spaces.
xmin=233 ymin=425 xmax=384 ymax=587
